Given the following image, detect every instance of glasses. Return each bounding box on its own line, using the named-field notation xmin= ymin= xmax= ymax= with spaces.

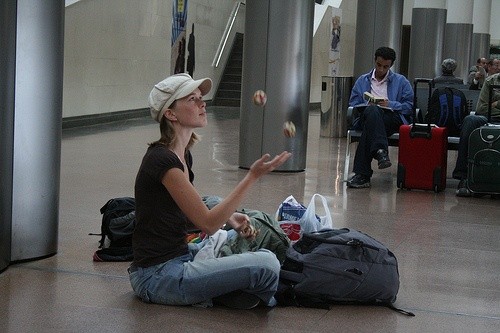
xmin=483 ymin=62 xmax=488 ymax=65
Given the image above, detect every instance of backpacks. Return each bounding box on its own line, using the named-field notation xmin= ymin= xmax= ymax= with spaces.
xmin=202 ymin=194 xmax=289 ymax=266
xmin=89 ymin=197 xmax=136 ymax=262
xmin=280 ymin=228 xmax=415 ymax=317
xmin=430 ymin=86 xmax=468 ymax=137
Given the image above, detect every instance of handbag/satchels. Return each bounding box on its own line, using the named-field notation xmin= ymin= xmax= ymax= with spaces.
xmin=275 ymin=193 xmax=333 ymax=246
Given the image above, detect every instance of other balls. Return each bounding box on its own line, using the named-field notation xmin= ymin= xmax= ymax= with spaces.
xmin=283 ymin=123 xmax=296 ymax=139
xmin=241 ymin=223 xmax=255 ymax=236
xmin=253 ymin=90 xmax=266 ymax=106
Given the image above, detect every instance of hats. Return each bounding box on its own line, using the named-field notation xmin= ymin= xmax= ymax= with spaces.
xmin=442 ymin=59 xmax=457 ymax=71
xmin=149 ymin=73 xmax=212 ymax=124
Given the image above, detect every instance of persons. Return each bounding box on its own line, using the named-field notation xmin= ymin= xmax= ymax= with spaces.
xmin=347 ymin=47 xmax=500 ymax=189
xmin=127 ymin=74 xmax=293 ymax=312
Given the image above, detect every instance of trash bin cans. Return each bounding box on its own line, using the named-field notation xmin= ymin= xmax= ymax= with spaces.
xmin=319 ymin=75 xmax=354 ymax=139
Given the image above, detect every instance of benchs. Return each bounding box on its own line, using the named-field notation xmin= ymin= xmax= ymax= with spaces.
xmin=341 ymin=86 xmax=480 ymax=183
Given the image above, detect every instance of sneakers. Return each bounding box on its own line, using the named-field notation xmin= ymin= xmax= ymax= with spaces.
xmin=377 ymin=150 xmax=391 ymax=169
xmin=347 ymin=174 xmax=371 ymax=188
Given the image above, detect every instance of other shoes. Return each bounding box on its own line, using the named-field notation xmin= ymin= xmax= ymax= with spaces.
xmin=213 ymin=290 xmax=260 ymax=309
xmin=456 ymin=180 xmax=470 ymax=196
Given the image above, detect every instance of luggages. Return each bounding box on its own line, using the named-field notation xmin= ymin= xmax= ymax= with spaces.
xmin=397 ymin=77 xmax=448 ymax=193
xmin=468 ymin=85 xmax=500 ymax=196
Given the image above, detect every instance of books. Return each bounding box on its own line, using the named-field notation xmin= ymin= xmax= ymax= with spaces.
xmin=363 ymin=91 xmax=385 ymax=104
